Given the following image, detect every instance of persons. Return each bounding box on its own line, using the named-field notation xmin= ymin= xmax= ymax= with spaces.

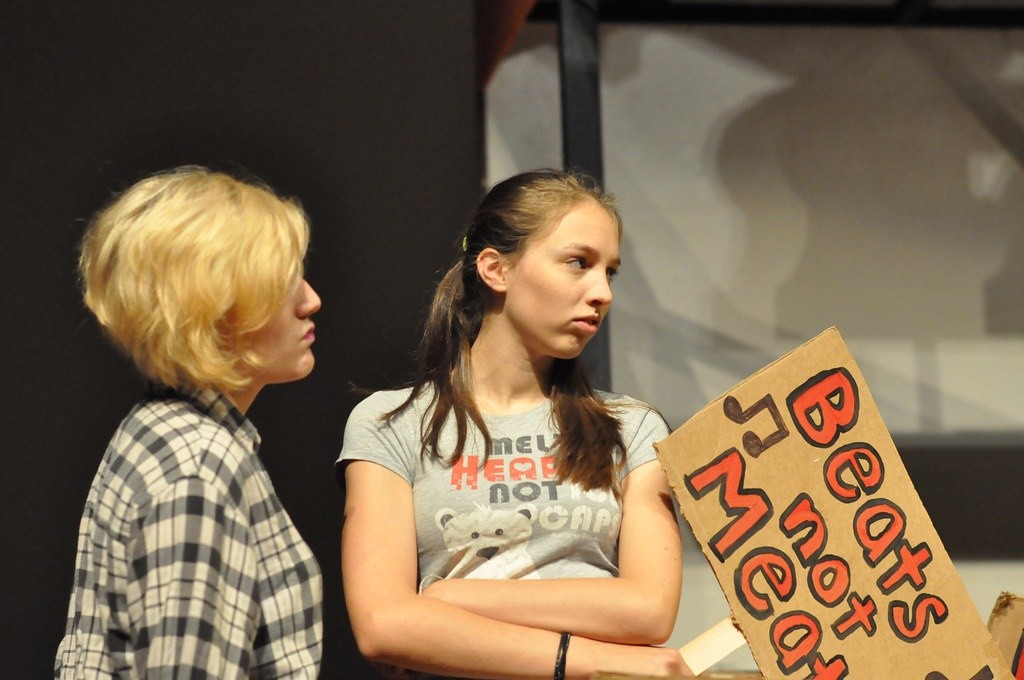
xmin=54 ymin=165 xmax=326 ymax=680
xmin=339 ymin=165 xmax=700 ymax=680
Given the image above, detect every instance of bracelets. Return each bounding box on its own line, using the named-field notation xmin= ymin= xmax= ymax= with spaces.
xmin=554 ymin=632 xmax=571 ymax=680
